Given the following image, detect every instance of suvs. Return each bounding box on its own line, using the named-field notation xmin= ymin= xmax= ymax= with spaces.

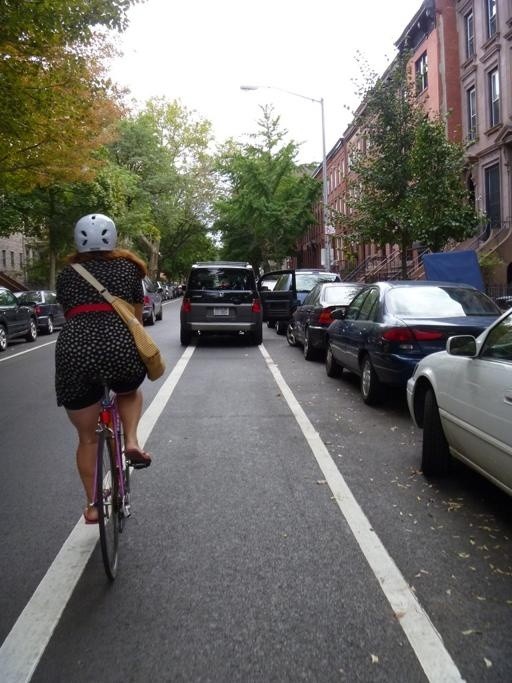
xmin=0 ymin=287 xmax=38 ymax=352
xmin=142 ymin=276 xmax=163 ymax=326
xmin=267 ymin=272 xmax=342 ymax=335
xmin=180 ymin=261 xmax=297 ymax=346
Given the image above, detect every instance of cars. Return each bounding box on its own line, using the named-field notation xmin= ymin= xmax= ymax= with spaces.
xmin=407 ymin=308 xmax=512 ymax=501
xmin=13 ymin=290 xmax=67 ymax=334
xmin=325 ymin=280 xmax=503 ymax=407
xmin=286 ymin=282 xmax=371 ymax=361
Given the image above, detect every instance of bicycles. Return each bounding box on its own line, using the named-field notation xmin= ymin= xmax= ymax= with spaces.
xmin=83 ymin=370 xmax=151 ymax=582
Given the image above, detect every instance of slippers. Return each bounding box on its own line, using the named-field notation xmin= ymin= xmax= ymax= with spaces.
xmin=83 ymin=508 xmax=98 ymax=523
xmin=125 ymin=449 xmax=152 ymax=467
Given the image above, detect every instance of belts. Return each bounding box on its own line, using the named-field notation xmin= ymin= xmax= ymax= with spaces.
xmin=64 ymin=304 xmax=113 ymax=320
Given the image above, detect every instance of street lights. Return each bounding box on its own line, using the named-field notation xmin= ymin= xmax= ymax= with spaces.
xmin=240 ymin=83 xmax=331 ymax=274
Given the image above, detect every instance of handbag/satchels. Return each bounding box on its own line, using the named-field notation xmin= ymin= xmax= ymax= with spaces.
xmin=69 ymin=261 xmax=166 ymax=381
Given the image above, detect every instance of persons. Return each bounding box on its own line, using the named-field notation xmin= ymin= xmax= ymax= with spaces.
xmin=55 ymin=214 xmax=151 ymax=524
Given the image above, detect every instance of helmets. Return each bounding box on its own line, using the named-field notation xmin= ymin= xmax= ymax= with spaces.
xmin=75 ymin=214 xmax=117 ymax=253
